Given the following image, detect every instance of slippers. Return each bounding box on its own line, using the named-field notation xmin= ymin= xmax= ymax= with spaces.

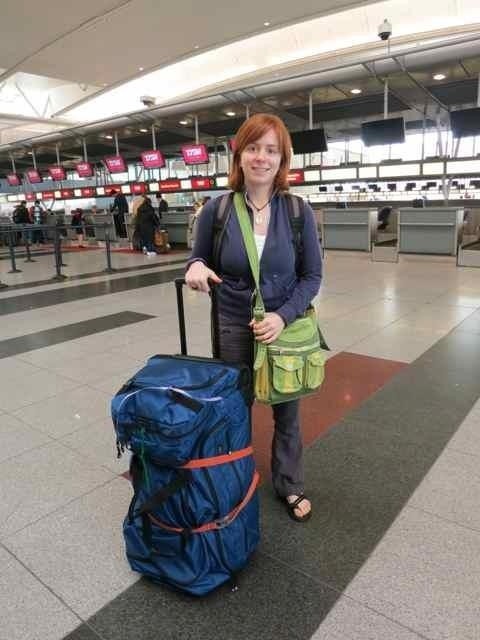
xmin=280 ymin=493 xmax=313 ymax=522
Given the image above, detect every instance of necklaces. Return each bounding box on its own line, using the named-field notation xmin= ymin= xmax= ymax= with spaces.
xmin=247 ymin=194 xmax=271 ymax=225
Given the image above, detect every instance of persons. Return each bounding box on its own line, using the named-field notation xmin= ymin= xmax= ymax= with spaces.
xmin=71 ymin=208 xmax=85 ymax=248
xmin=190 ymin=196 xmax=211 ymax=250
xmin=13 ymin=200 xmax=46 ymax=247
xmin=111 ymin=189 xmax=168 ymax=256
xmin=185 ymin=113 xmax=322 ymax=522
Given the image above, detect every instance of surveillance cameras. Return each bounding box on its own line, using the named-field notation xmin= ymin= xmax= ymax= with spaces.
xmin=378 ymin=22 xmax=392 ymax=40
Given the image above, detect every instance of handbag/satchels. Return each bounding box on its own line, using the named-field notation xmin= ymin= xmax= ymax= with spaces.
xmin=251 ymin=311 xmax=324 ymax=408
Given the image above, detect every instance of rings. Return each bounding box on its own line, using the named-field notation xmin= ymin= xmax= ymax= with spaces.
xmin=255 ymin=324 xmax=257 ymax=330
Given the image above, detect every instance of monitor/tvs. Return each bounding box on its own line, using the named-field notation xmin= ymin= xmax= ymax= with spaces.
xmin=75 ymin=163 xmax=94 ymax=177
xmin=362 ymin=117 xmax=404 ymax=146
xmin=181 ymin=144 xmax=209 ymax=165
xmin=450 ymin=107 xmax=480 ymax=137
xmin=139 ymin=150 xmax=165 ymax=169
xmin=104 ymin=156 xmax=128 ymax=173
xmin=26 ymin=170 xmax=42 ymax=183
xmin=228 ymin=139 xmax=236 ymax=152
xmin=48 ymin=166 xmax=66 ymax=181
xmin=290 ymin=128 xmax=327 ymax=154
xmin=5 ymin=173 xmax=22 ymax=185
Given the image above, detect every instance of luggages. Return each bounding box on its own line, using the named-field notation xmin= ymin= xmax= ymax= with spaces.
xmin=108 ymin=276 xmax=263 ymax=598
